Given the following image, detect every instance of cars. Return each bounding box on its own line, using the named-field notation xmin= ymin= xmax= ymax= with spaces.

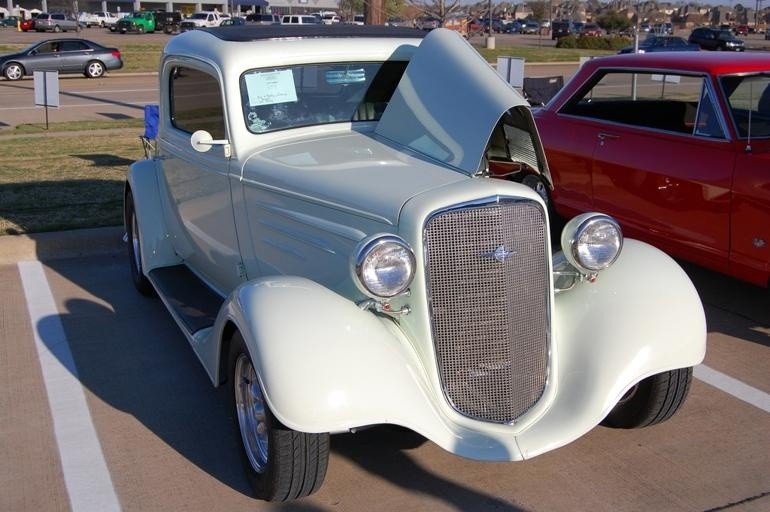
xmin=33 ymin=11 xmax=82 ymax=33
xmin=615 ymin=34 xmax=704 ymax=54
xmin=413 ymin=12 xmax=769 ymax=39
xmin=0 ymin=37 xmax=123 ymax=80
xmin=481 ymin=50 xmax=770 ymax=303
xmin=0 ymin=15 xmax=26 ymax=28
xmin=20 ymin=18 xmax=38 ymax=32
xmin=86 ymin=11 xmax=120 ymax=29
xmin=123 ymin=22 xmax=709 ymax=504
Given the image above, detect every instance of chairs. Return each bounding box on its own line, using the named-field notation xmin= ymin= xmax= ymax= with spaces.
xmin=523 ymin=76 xmax=564 ymax=107
xmin=333 ymin=83 xmax=382 ymax=122
xmin=140 ymin=105 xmax=159 ymax=158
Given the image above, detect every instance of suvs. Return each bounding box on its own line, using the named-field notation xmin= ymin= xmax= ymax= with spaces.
xmin=688 ymin=27 xmax=747 ymax=51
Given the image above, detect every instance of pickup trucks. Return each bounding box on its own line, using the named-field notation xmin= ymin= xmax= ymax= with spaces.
xmin=109 ymin=8 xmax=407 ymax=33
